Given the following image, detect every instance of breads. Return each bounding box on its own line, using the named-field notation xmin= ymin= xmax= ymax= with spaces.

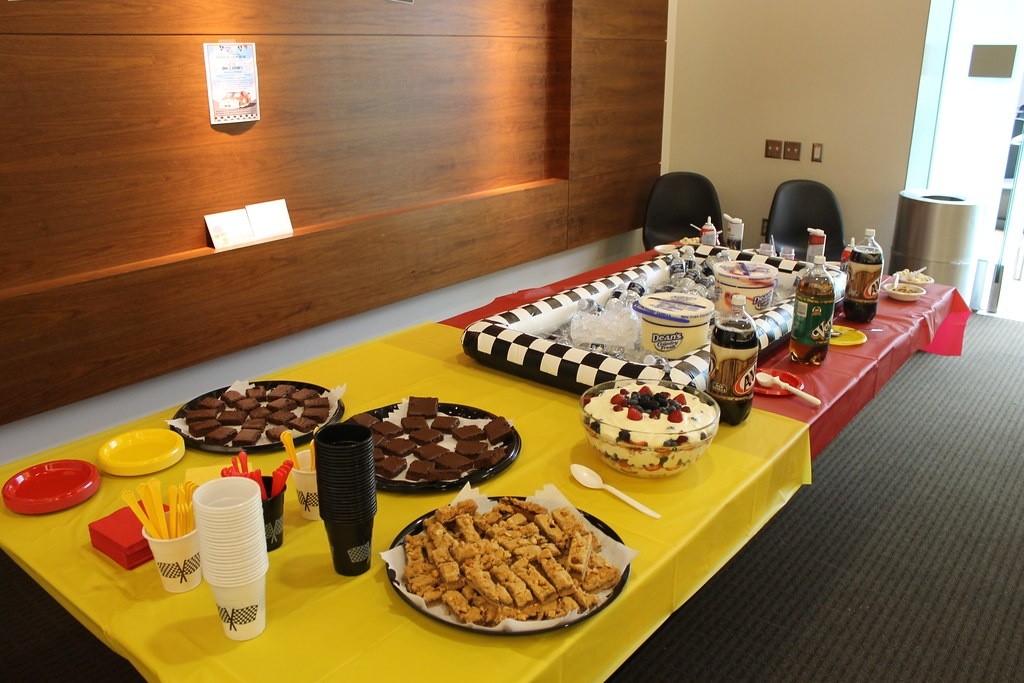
xmin=405 ymin=498 xmax=621 ymax=626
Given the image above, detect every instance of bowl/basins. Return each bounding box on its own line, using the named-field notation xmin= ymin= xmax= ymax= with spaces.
xmin=578 ymin=379 xmax=721 ymax=479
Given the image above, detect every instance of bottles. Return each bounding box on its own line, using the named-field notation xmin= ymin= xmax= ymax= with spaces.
xmin=708 ymin=295 xmax=759 ymax=427
xmin=840 ymin=228 xmax=885 ymax=324
xmin=699 ymin=214 xmax=857 ymax=271
xmin=788 ymin=256 xmax=835 ymax=367
xmin=555 ymin=247 xmax=779 ymax=387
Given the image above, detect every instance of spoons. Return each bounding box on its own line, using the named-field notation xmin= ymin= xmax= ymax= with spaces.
xmin=756 ymin=372 xmax=823 ymax=407
xmin=570 ymin=463 xmax=663 ymax=520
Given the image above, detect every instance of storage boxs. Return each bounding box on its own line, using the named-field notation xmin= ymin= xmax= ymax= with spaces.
xmin=89 ymin=500 xmax=169 ymax=571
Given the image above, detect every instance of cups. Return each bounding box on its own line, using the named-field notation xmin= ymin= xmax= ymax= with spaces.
xmin=261 ymin=475 xmax=287 ymax=552
xmin=314 ymin=423 xmax=378 ymax=577
xmin=290 ymin=452 xmax=323 ymax=522
xmin=141 ymin=512 xmax=202 ymax=593
xmin=194 ymin=477 xmax=268 ymax=640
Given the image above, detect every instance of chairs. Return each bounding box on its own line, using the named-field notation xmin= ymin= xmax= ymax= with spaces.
xmin=642 ymin=172 xmax=729 ymax=251
xmin=766 ymin=180 xmax=848 ymax=262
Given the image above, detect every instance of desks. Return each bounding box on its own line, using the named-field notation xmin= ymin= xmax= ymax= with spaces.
xmin=1 ymin=238 xmax=970 ymax=683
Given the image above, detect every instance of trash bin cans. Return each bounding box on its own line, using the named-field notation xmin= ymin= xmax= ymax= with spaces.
xmin=888 ymin=190 xmax=980 ymax=307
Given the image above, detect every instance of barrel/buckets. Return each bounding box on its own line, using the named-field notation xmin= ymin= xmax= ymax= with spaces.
xmin=710 ymin=262 xmax=778 ymax=316
xmin=633 ymin=292 xmax=713 ymax=362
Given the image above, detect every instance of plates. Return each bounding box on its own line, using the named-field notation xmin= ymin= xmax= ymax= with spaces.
xmin=2 ymin=459 xmax=102 ymax=515
xmin=387 ymin=495 xmax=630 ymax=635
xmin=886 ymin=271 xmax=934 ymax=302
xmin=829 ymin=325 xmax=868 ymax=345
xmin=172 ymin=381 xmax=344 ymax=454
xmin=99 ymin=429 xmax=185 ymax=476
xmin=345 ymin=400 xmax=521 ymax=491
xmin=753 ymin=368 xmax=804 ymax=397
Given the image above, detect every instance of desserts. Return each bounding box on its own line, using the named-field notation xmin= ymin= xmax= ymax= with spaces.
xmin=582 ymin=383 xmax=714 ymax=479
xmin=347 ymin=396 xmax=513 ymax=482
xmin=185 ymin=384 xmax=331 ymax=446
